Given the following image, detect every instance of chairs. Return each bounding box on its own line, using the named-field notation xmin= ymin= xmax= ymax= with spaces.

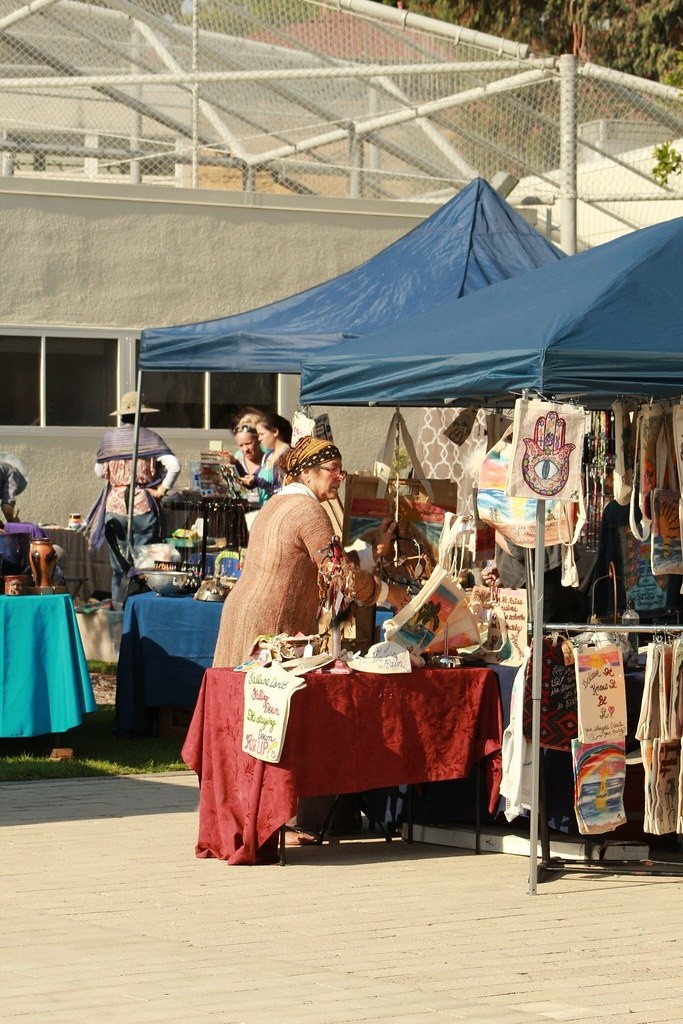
xmin=4 ymin=522 xmax=48 ymax=538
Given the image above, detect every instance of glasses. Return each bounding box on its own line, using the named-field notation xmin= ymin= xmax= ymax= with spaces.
xmin=233 ymin=425 xmax=256 ymax=435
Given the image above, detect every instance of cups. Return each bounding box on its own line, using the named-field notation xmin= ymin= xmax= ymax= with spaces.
xmin=4 ymin=575 xmax=27 ymax=596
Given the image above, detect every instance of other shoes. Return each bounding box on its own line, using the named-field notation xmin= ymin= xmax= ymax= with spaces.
xmin=276 ymin=825 xmax=316 ymax=846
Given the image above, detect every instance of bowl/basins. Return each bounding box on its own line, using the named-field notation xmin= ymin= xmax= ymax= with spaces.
xmin=142 ymin=571 xmax=188 ymax=597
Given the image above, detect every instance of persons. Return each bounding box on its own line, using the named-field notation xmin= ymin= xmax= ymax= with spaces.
xmin=95 ymin=390 xmax=181 ymax=609
xmin=211 ymin=408 xmax=412 ymax=668
xmin=0 ymin=463 xmax=28 ymax=531
xmin=493 ymin=528 xmax=582 ymax=624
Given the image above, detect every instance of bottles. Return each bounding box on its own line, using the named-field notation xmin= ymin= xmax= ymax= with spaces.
xmin=622 ymin=600 xmax=639 ymax=668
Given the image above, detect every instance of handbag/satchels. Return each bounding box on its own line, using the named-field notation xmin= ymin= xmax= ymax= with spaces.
xmin=504 ymin=398 xmax=589 ymax=546
xmin=475 ymin=424 xmax=570 ymax=549
xmin=628 ymin=398 xmax=683 ymax=577
xmin=383 ymin=511 xmax=507 ymax=657
xmin=241 ymin=650 xmax=333 ymax=765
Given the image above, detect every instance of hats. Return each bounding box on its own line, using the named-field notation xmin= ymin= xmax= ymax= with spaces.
xmin=107 ymin=390 xmax=161 ymax=417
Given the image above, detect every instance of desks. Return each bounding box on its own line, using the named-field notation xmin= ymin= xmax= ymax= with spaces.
xmin=0 ymin=592 xmax=98 ymax=758
xmin=161 ymin=495 xmax=262 ymax=566
xmin=181 ymin=665 xmax=503 ymax=868
xmin=39 ymin=524 xmax=113 ymax=594
xmin=112 ymin=591 xmax=224 ymax=738
xmin=522 ymin=655 xmax=651 ymax=863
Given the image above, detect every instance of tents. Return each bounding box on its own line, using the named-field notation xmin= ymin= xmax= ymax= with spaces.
xmin=124 ymin=178 xmax=569 ymax=566
xmin=300 ymin=215 xmax=683 ymax=893
